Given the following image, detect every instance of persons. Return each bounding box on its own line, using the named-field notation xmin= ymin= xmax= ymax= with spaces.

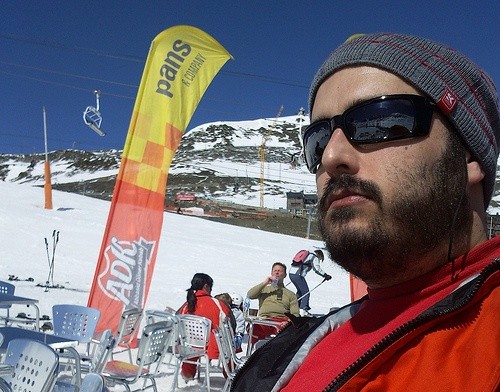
xmin=247 ymin=262 xmax=300 ymax=319
xmin=175 ymin=273 xmax=236 ymax=379
xmin=289 ymin=250 xmax=332 ymax=310
xmin=229 ymin=31 xmax=500 ymax=392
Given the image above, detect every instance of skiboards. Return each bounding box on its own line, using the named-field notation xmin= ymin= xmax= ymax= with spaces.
xmin=36 ymin=283 xmax=89 ymax=292
xmin=8 ymin=275 xmax=34 ymax=281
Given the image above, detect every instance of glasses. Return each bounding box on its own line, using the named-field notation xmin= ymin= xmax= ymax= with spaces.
xmin=303 ymin=94 xmax=441 ymax=173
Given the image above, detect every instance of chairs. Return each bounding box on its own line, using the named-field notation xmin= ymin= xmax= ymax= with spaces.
xmin=0 ymin=281 xmax=290 ymax=392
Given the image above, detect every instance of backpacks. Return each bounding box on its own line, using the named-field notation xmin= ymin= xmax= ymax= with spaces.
xmin=291 ymin=250 xmax=319 ymax=266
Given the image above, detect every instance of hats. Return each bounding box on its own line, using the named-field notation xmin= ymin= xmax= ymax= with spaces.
xmin=309 ymin=33 xmax=500 ymax=211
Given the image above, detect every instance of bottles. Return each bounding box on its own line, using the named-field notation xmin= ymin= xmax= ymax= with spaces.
xmin=243 ymin=295 xmax=251 ymax=317
xmin=271 ymin=276 xmax=279 ymax=289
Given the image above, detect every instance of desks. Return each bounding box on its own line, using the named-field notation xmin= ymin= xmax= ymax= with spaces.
xmin=0 ymin=327 xmax=81 ymax=392
xmin=0 ymin=295 xmax=40 ymax=333
